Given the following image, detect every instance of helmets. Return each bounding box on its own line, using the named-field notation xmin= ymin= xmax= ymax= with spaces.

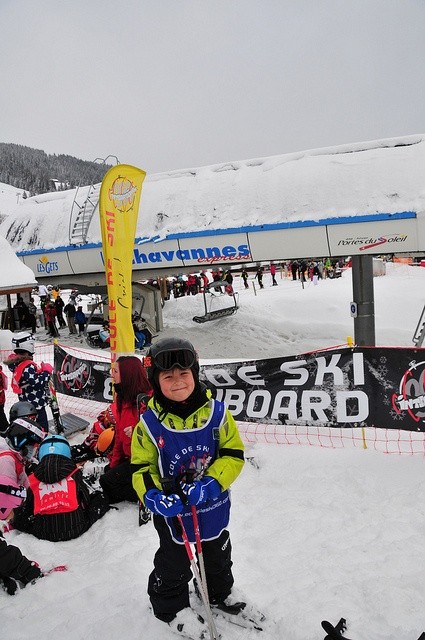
xmin=9 ymin=401 xmax=38 ymax=422
xmin=8 ymin=417 xmax=45 ymax=451
xmin=38 ymin=435 xmax=71 ymax=462
xmin=95 ymin=427 xmax=115 ymax=456
xmin=0 ymin=474 xmax=27 ymax=520
xmin=148 ymin=337 xmax=199 ymax=384
xmin=11 ymin=331 xmax=35 ymax=354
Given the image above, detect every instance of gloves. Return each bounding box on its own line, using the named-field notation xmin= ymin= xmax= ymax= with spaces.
xmin=40 ymin=361 xmax=54 ymax=374
xmin=144 ymin=488 xmax=183 ymax=517
xmin=19 ymin=557 xmax=44 ymax=585
xmin=181 ymin=476 xmax=221 ymax=505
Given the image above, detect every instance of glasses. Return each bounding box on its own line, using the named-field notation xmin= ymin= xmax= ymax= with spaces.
xmin=0 ymin=484 xmax=27 ymax=503
xmin=151 ymin=349 xmax=196 ymax=371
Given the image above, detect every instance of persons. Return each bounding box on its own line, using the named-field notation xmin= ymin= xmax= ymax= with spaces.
xmin=241 ymin=269 xmax=249 ymax=289
xmin=69 ymin=406 xmax=116 ymax=463
xmin=15 ymin=297 xmax=30 ymax=328
xmin=20 ymin=435 xmax=108 ymax=540
xmin=9 ymin=401 xmax=36 ymax=426
xmin=148 ymin=269 xmax=233 ymax=300
xmin=298 ymin=258 xmax=343 ymax=279
xmin=130 ymin=338 xmax=245 ymax=640
xmin=0 ymin=418 xmax=45 ymax=523
xmin=255 ymin=268 xmax=264 ymax=289
xmin=292 ymin=261 xmax=298 ymax=280
xmin=38 ymin=285 xmax=110 ymax=348
xmin=0 ymin=365 xmax=10 ymax=435
xmin=6 ymin=340 xmax=53 ymax=432
xmin=28 ymin=298 xmax=37 ymax=333
xmin=101 ymin=356 xmax=150 ymax=503
xmin=271 ymin=264 xmax=278 ymax=286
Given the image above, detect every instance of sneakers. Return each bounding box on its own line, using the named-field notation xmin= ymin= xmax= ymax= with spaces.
xmin=169 ymin=607 xmax=209 ymax=640
xmin=211 ymin=591 xmax=247 ymax=618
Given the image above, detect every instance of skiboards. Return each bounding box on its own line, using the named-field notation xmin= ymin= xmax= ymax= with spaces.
xmin=48 ymin=374 xmax=67 ymax=438
